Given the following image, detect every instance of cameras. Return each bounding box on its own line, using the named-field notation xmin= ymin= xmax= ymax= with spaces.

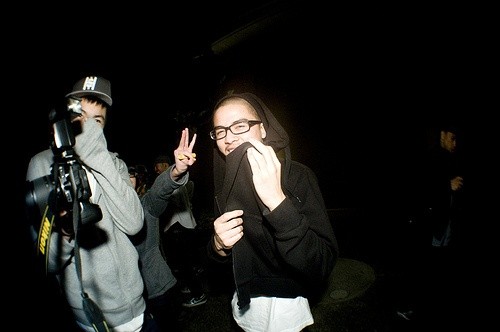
xmin=45 ymin=107 xmax=102 ymax=234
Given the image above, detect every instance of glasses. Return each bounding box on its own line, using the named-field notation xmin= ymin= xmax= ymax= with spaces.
xmin=211 ymin=120 xmax=263 ymax=140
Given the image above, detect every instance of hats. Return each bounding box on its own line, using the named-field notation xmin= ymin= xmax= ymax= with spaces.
xmin=66 ymin=76 xmax=112 ymax=107
xmin=152 ymin=156 xmax=171 ymax=163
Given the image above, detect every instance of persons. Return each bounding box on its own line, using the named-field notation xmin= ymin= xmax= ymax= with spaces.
xmin=392 ymin=125 xmax=465 ymax=319
xmin=25 ymin=76 xmax=146 ymax=332
xmin=129 ymin=128 xmax=197 ymax=332
xmin=206 ymin=92 xmax=340 ymax=332
xmin=152 ymin=152 xmax=208 ymax=307
